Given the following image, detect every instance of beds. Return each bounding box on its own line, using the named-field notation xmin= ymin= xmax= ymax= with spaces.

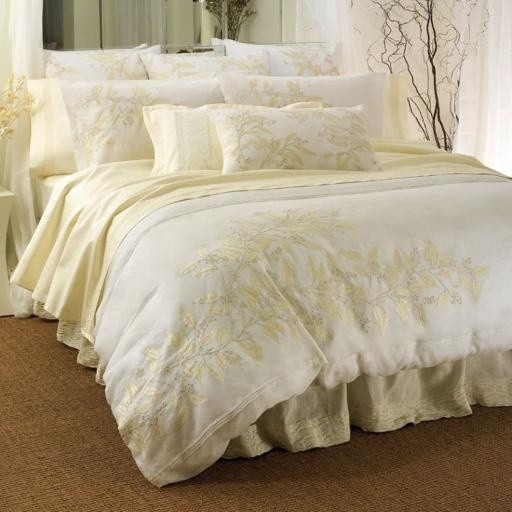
xmin=15 ymin=39 xmax=512 ymax=487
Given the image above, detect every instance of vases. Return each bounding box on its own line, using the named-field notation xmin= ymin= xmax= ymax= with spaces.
xmin=0 ymin=139 xmax=9 ymax=191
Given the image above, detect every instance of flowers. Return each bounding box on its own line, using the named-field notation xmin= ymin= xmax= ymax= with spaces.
xmin=0 ymin=73 xmax=34 ymax=140
